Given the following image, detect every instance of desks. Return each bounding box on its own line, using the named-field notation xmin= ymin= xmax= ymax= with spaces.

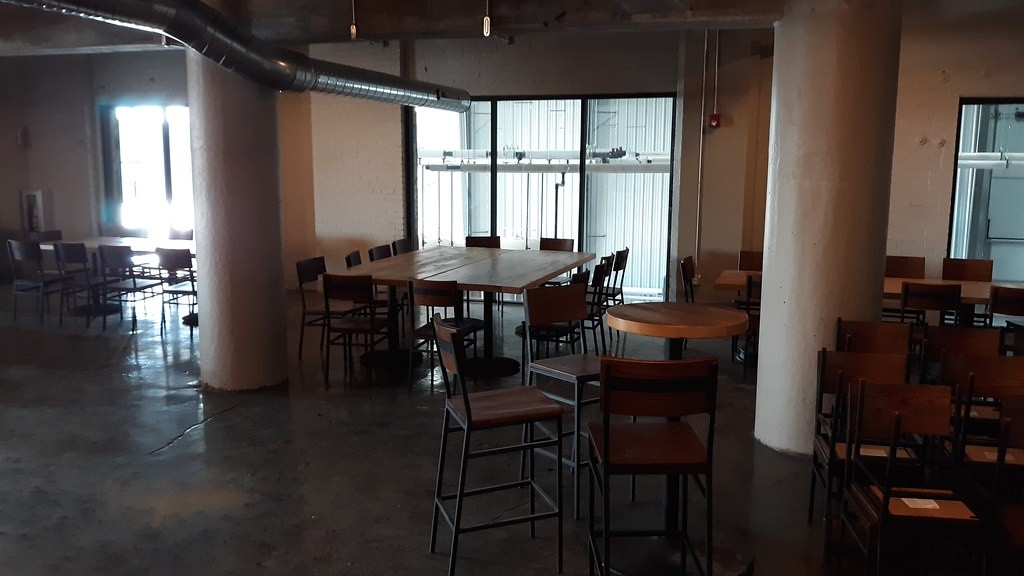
xmin=38 ymin=237 xmax=197 ymax=315
xmin=324 ymin=244 xmax=597 ymax=357
xmin=712 ymin=269 xmax=1024 ymax=331
xmin=602 ymin=300 xmax=749 ymax=421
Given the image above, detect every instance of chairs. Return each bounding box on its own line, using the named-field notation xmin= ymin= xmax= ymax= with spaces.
xmin=6 ymin=230 xmax=200 ymax=339
xmin=295 ymin=235 xmax=1024 ymax=576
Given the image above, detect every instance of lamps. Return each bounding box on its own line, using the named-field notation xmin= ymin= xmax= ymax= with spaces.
xmin=483 ymin=1 xmax=491 ymax=37
xmin=349 ymin=1 xmax=356 ymax=42
